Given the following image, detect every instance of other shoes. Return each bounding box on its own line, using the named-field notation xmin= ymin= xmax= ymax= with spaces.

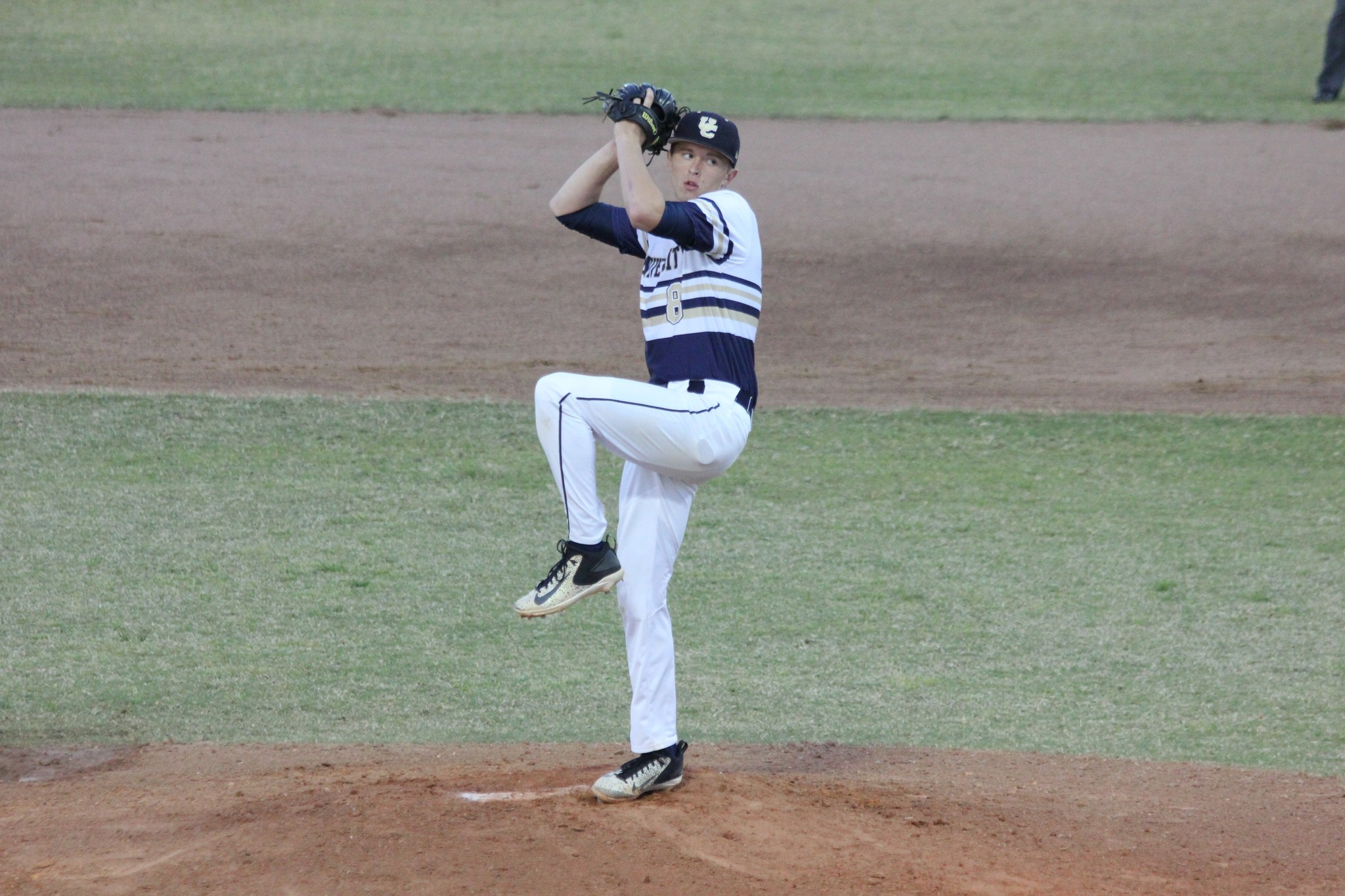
xmin=1313 ymin=89 xmax=1339 ymax=105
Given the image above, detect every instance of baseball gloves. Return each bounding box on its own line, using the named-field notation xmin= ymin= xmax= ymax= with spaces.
xmin=601 ymin=82 xmax=682 ymax=156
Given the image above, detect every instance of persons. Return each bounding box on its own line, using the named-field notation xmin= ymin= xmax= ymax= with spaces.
xmin=513 ymin=77 xmax=763 ymax=803
xmin=1314 ymin=0 xmax=1345 ymax=102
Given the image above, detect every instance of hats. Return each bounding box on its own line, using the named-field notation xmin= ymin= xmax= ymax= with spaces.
xmin=667 ymin=111 xmax=740 ymax=169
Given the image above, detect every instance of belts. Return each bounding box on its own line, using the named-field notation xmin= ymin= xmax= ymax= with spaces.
xmin=687 ymin=381 xmax=753 ymax=412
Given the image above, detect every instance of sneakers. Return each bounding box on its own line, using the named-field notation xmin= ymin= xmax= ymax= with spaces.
xmin=513 ymin=535 xmax=624 ymax=621
xmin=592 ymin=740 xmax=688 ymax=804
